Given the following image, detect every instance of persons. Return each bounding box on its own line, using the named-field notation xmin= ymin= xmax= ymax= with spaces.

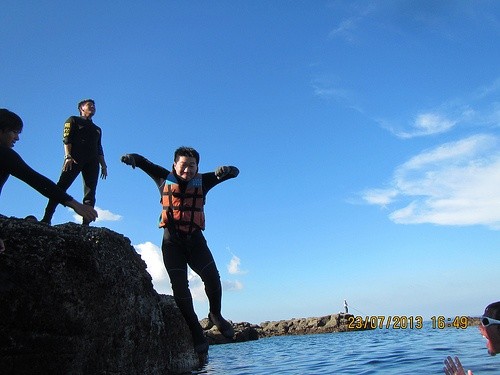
xmin=344 ymin=300 xmax=349 ymax=313
xmin=120 ymin=145 xmax=239 ymax=353
xmin=0 ymin=109 xmax=97 ymax=354
xmin=39 ymin=98 xmax=108 ymax=226
xmin=443 ymin=301 xmax=500 ymax=375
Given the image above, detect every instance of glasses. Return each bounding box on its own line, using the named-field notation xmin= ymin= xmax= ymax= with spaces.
xmin=480 ymin=317 xmax=500 ymax=327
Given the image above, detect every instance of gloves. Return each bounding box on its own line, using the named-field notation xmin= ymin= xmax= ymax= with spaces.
xmin=62 ymin=154 xmax=77 ymax=171
xmin=120 ymin=154 xmax=136 ymax=169
xmin=215 ymin=165 xmax=230 ymax=180
xmin=100 ymin=165 xmax=108 ymax=179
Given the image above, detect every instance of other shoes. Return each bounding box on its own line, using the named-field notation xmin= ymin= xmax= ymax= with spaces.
xmin=209 ymin=312 xmax=235 ymax=338
xmin=191 ymin=326 xmax=210 ymax=353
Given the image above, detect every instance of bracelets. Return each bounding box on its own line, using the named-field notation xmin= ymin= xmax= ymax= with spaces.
xmin=64 ymin=154 xmax=72 ymax=159
